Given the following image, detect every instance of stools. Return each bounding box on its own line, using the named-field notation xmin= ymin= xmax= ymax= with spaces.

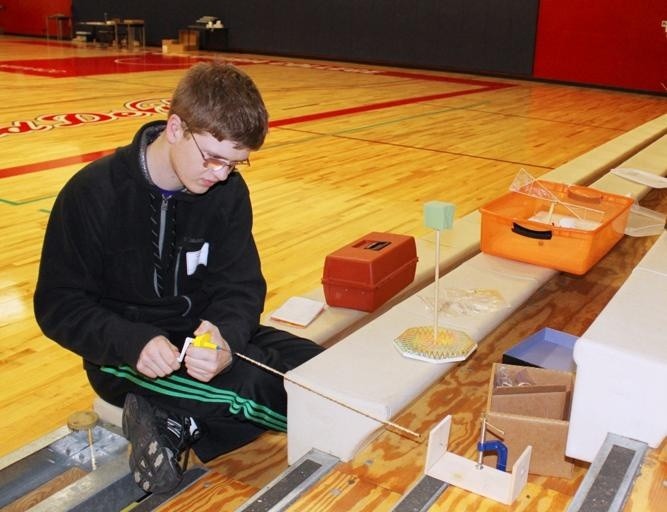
xmin=45 ymin=13 xmax=70 ymax=42
xmin=124 ymin=19 xmax=145 ymax=51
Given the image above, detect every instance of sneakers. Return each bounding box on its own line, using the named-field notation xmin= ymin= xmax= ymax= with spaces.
xmin=120 ymin=392 xmax=194 ymax=494
xmin=175 ymin=114 xmax=252 ymax=172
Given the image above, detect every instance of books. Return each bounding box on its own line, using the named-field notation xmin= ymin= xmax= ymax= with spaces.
xmin=269 ymin=291 xmax=327 ymax=331
xmin=34 ymin=64 xmax=327 ymax=495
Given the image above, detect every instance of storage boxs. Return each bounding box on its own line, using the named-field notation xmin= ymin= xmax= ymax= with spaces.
xmin=482 ymin=361 xmax=575 ymax=480
xmin=321 ymin=231 xmax=419 ymax=311
xmin=478 ymin=180 xmax=634 ymax=275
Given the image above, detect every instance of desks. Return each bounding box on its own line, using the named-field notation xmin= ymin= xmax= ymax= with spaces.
xmin=177 ymin=23 xmax=232 ymax=52
